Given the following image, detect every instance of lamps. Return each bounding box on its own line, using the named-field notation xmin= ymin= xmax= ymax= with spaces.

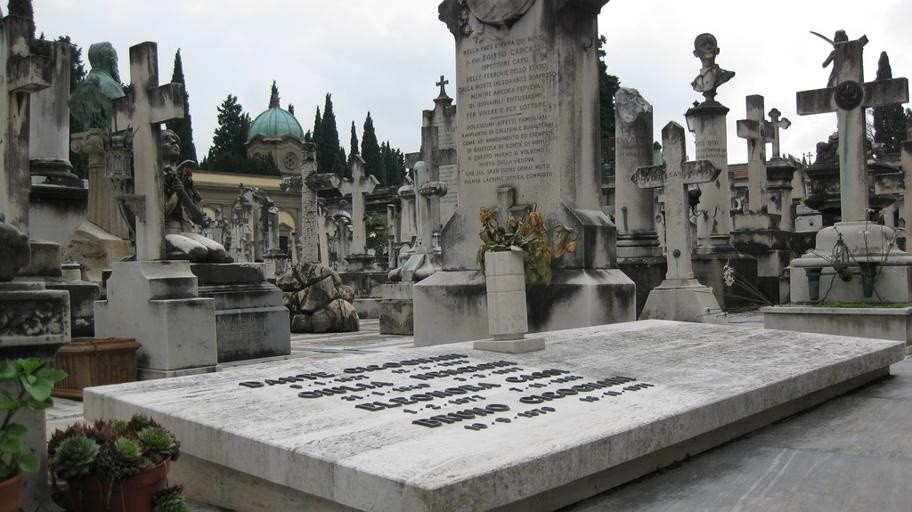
xmin=827 ymin=232 xmax=852 ymax=280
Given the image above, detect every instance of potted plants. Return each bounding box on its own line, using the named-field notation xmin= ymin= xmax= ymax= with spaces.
xmin=1 ymin=355 xmax=185 ymax=511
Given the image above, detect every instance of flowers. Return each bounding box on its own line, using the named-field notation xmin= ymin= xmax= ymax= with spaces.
xmin=476 ymin=203 xmax=578 ymax=283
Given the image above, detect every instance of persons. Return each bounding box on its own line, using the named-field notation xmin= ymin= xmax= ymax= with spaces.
xmin=682 ymin=33 xmax=733 ymax=95
xmin=162 ymin=132 xmax=231 ymax=264
xmin=65 ymin=40 xmax=129 ymax=133
